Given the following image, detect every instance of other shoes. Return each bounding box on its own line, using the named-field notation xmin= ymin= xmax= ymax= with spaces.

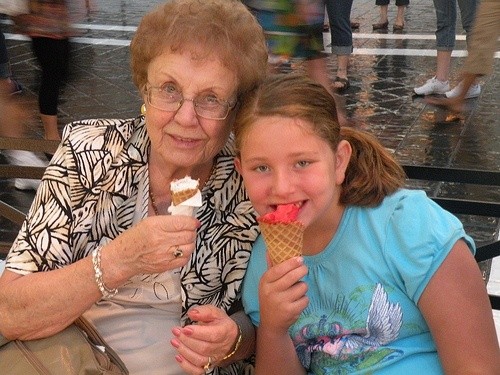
xmin=323 ymin=25 xmax=329 ymax=31
xmin=373 ymin=19 xmax=388 ymax=29
xmin=14 ymin=178 xmax=41 ymax=191
xmin=351 ymin=22 xmax=359 ymax=28
xmin=393 ymin=23 xmax=404 ymax=29
xmin=44 ymin=151 xmax=54 ymax=161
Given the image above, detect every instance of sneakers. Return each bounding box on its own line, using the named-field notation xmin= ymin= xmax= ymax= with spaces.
xmin=445 ymin=81 xmax=480 ymax=99
xmin=413 ymin=75 xmax=450 ymax=95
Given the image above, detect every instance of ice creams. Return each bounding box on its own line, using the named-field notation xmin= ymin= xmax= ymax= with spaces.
xmin=170 ymin=174 xmax=200 ymax=206
xmin=257 ymin=204 xmax=304 ymax=267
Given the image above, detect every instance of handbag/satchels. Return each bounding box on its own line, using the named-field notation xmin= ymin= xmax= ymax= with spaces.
xmin=0 ymin=312 xmax=129 ymax=375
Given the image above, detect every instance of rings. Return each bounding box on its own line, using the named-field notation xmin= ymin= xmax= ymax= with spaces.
xmin=173 ymin=245 xmax=183 ymax=259
xmin=203 ymin=356 xmax=213 ymax=370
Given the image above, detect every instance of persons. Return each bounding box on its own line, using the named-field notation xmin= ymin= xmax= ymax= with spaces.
xmin=0 ymin=0 xmax=269 ymax=375
xmin=233 ymin=73 xmax=500 ymax=375
xmin=0 ymin=0 xmax=500 ymax=191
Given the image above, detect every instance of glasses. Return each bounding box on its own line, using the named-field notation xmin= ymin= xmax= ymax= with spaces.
xmin=144 ymin=82 xmax=239 ymax=120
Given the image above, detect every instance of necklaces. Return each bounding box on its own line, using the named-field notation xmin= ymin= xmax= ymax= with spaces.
xmin=149 ymin=162 xmax=217 ymax=216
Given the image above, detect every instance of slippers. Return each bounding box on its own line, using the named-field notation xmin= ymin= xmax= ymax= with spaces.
xmin=333 ymin=77 xmax=349 ymax=91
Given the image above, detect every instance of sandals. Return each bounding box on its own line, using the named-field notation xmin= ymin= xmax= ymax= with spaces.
xmin=421 ymin=107 xmax=466 ymax=126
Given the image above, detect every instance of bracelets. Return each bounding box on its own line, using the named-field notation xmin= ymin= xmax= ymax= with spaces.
xmin=92 ymin=246 xmax=118 ymax=298
xmin=221 ymin=325 xmax=243 ymax=360
